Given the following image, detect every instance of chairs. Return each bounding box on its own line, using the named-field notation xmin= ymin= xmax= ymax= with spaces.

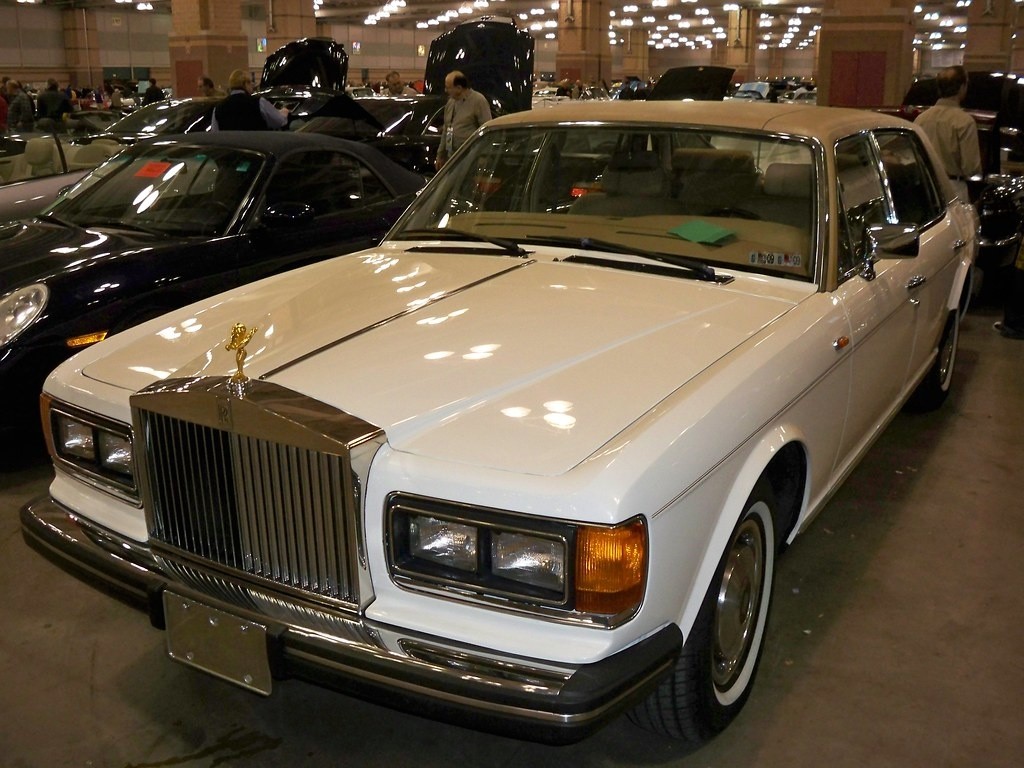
xmin=212 ymin=171 xmax=276 ymax=217
xmin=68 ymin=143 xmax=112 ymax=172
xmin=565 ymin=147 xmax=688 ymax=217
xmin=732 ymin=162 xmax=815 ymax=232
xmin=9 ymin=137 xmax=61 ymax=181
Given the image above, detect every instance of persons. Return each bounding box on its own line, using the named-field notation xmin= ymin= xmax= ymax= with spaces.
xmin=380 ymin=71 xmax=416 ymax=96
xmin=556 ymin=78 xmax=588 ymax=99
xmin=0 ymin=76 xmax=36 ymax=133
xmin=66 ymin=84 xmax=71 ymax=97
xmin=197 ymin=77 xmax=226 ymax=96
xmin=434 ymin=71 xmax=494 ymax=198
xmin=766 ymin=84 xmax=779 ymax=103
xmin=992 ymin=237 xmax=1024 ymax=340
xmin=36 ymin=78 xmax=74 ymax=132
xmin=210 ymin=68 xmax=290 ymax=131
xmin=620 ymin=80 xmax=654 ymax=100
xmin=145 ymin=78 xmax=165 ymax=104
xmin=913 ymin=65 xmax=981 ymax=203
xmin=75 ymin=80 xmax=129 ymax=102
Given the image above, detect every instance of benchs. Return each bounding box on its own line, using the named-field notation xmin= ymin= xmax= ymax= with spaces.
xmin=665 ymin=148 xmax=930 ymax=218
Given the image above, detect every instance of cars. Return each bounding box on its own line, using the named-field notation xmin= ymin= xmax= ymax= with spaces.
xmin=17 ymin=98 xmax=979 ymax=747
xmin=0 ymin=14 xmax=1023 ymax=467
